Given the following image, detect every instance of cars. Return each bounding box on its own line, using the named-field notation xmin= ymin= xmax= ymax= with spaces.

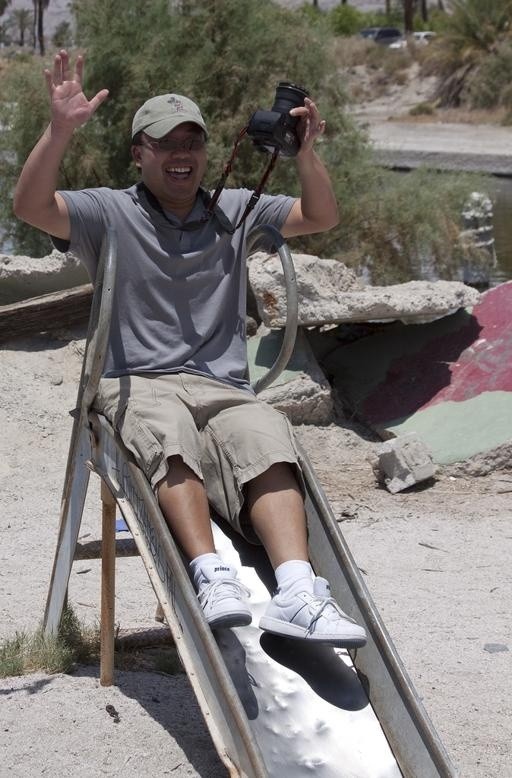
xmin=361 ymin=28 xmax=402 ymax=45
xmin=390 ymin=31 xmax=437 ymax=49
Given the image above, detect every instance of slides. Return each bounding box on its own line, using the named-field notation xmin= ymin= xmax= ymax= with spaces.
xmin=85 ymin=410 xmax=458 ymax=777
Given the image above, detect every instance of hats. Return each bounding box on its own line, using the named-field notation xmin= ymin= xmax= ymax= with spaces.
xmin=130 ymin=93 xmax=211 ymax=143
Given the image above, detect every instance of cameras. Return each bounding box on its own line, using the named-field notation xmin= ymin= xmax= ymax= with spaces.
xmin=245 ymin=82 xmax=311 ymax=159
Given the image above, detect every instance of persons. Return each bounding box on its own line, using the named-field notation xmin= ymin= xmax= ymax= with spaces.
xmin=14 ymin=49 xmax=368 ymax=646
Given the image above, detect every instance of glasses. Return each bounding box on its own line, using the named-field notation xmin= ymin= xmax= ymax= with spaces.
xmin=139 ymin=136 xmax=209 ymax=153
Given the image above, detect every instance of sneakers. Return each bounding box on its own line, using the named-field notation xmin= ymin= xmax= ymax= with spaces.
xmin=257 ymin=574 xmax=369 ymax=654
xmin=191 ymin=561 xmax=255 ymax=631
